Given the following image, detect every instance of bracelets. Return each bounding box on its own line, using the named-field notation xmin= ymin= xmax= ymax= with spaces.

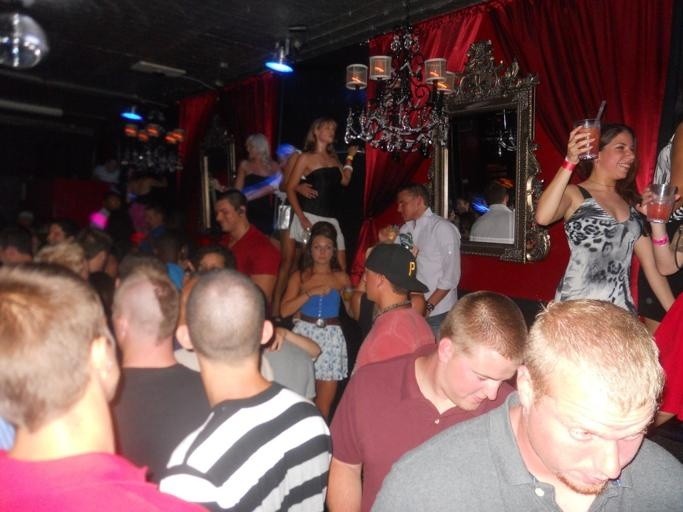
xmin=561 ymin=159 xmax=576 ymax=171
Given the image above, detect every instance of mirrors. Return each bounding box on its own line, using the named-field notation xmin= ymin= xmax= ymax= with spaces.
xmin=200 ymin=134 xmax=237 ymax=229
xmin=436 ymin=39 xmax=551 ymax=265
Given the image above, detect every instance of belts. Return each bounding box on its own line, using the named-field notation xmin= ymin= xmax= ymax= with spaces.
xmin=299 ymin=312 xmax=340 ymax=330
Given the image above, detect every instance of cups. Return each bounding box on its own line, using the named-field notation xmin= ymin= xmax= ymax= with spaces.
xmin=646 ymin=184 xmax=677 ymax=223
xmin=573 ymin=119 xmax=601 ymax=160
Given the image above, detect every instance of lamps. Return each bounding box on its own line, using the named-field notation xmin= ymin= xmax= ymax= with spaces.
xmin=263 ymin=41 xmax=293 ymax=74
xmin=343 ymin=26 xmax=455 ymax=162
xmin=488 ymin=106 xmax=518 ymax=156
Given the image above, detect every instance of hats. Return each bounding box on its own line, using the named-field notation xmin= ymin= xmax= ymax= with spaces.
xmin=363 ymin=242 xmax=428 ymax=292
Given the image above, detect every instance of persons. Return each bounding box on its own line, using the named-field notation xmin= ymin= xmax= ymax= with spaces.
xmin=472 ymin=180 xmax=516 ymax=245
xmin=635 ymin=107 xmax=683 ymax=425
xmin=534 ymin=123 xmax=676 ymax=318
